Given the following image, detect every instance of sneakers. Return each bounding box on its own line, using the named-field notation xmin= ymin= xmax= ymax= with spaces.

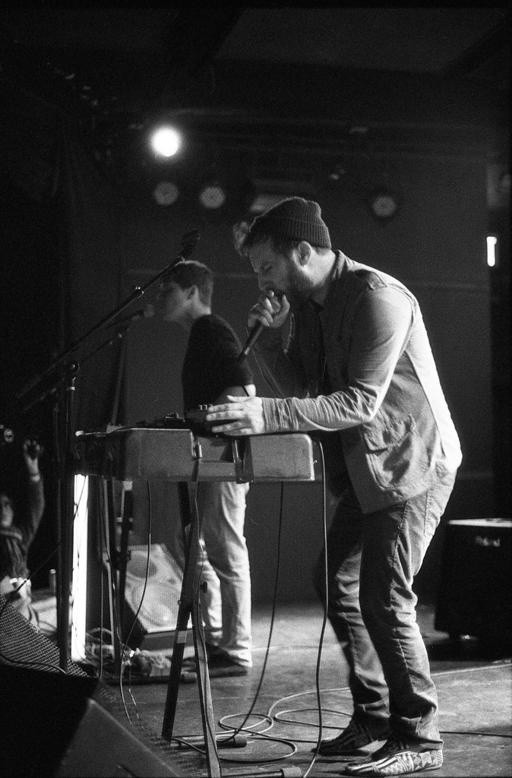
xmin=182 ymin=644 xmax=251 ymax=678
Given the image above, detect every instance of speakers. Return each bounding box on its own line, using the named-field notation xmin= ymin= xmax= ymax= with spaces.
xmin=111 ymin=543 xmax=196 ymax=651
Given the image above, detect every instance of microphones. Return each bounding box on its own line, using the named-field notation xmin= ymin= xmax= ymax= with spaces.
xmin=237 ymin=287 xmax=285 ymax=366
xmin=106 ymin=303 xmax=156 ymax=330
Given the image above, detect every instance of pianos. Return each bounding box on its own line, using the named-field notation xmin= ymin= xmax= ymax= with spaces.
xmin=73 ymin=418 xmax=316 ymax=488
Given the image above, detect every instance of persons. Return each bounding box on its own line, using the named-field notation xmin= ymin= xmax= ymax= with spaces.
xmin=206 ymin=197 xmax=464 ymax=776
xmin=158 ymin=259 xmax=256 ymax=678
xmin=0 ymin=439 xmax=44 ymax=630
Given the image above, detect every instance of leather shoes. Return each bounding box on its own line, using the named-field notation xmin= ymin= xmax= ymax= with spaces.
xmin=319 ymin=720 xmax=444 ymax=776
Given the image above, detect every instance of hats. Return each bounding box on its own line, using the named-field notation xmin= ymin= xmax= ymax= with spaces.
xmin=249 ymin=196 xmax=332 ymax=248
